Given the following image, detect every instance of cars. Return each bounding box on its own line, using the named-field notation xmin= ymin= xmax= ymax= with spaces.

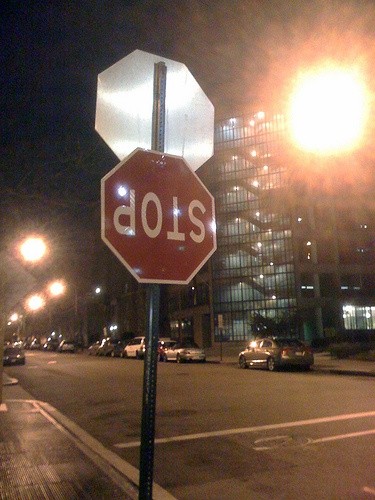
xmin=30 ymin=341 xmax=41 ymax=350
xmin=111 ymin=340 xmax=132 ymax=358
xmin=143 ymin=341 xmax=175 ymax=361
xmin=88 ymin=341 xmax=102 ymax=356
xmin=58 ymin=340 xmax=75 ymax=353
xmin=2 ymin=347 xmax=26 ymax=366
xmin=238 ymin=339 xmax=313 ymax=371
xmin=43 ymin=342 xmax=55 ymax=351
xmin=164 ymin=342 xmax=205 ymax=363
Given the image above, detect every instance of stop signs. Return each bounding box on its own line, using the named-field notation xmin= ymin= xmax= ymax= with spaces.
xmin=101 ymin=146 xmax=218 ymax=284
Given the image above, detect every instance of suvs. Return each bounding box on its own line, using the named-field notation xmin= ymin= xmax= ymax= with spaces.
xmin=123 ymin=337 xmax=162 ymax=360
xmin=98 ymin=339 xmax=120 ymax=356
